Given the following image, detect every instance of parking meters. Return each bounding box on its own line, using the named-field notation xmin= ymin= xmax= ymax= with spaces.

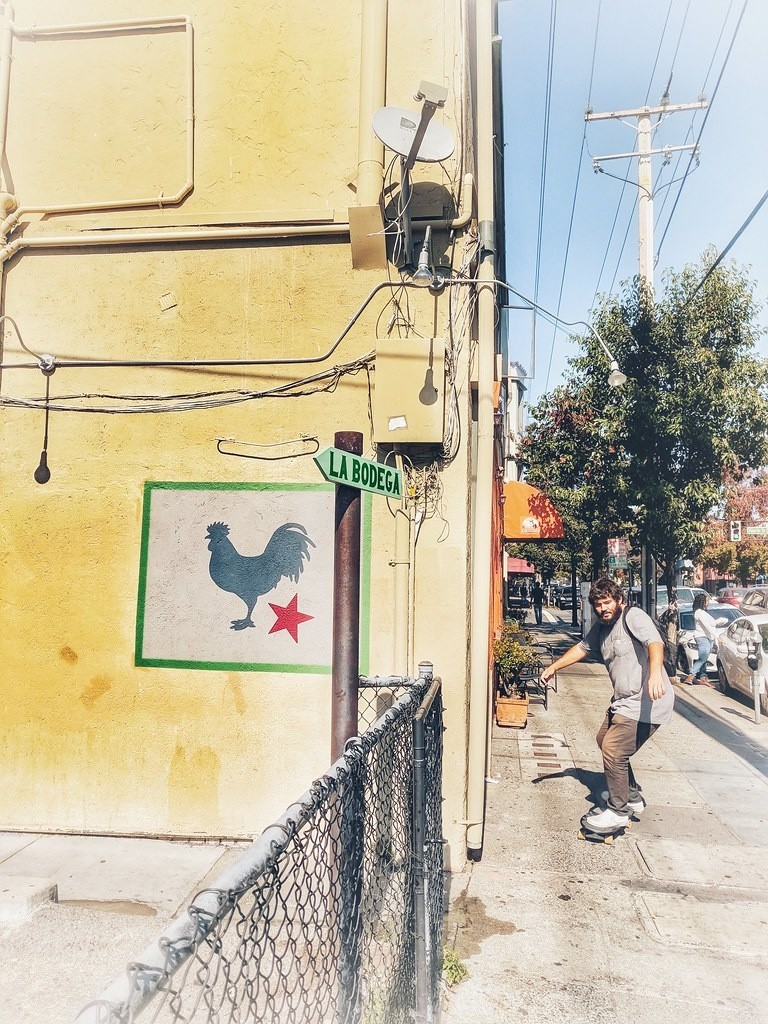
xmin=745 ymin=630 xmax=763 ymax=726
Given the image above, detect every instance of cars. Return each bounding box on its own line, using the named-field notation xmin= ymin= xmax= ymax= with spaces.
xmin=543 ymin=582 xmax=581 ymax=610
xmin=627 ymin=584 xmax=768 ymax=621
xmin=716 ymin=614 xmax=768 ymax=717
xmin=657 ymin=602 xmax=748 ymax=680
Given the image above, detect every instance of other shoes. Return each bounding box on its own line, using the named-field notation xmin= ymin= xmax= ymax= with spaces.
xmin=684 ymin=674 xmax=694 ymax=684
xmin=700 ymin=677 xmax=716 ymax=689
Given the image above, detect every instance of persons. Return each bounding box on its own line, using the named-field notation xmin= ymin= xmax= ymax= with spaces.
xmin=541 ymin=577 xmax=675 ymax=834
xmin=509 ymin=578 xmax=546 ymax=627
xmin=683 ymin=594 xmax=728 ymax=689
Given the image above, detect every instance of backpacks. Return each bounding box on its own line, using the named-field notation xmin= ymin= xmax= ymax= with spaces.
xmin=621 ymin=605 xmax=676 ymax=677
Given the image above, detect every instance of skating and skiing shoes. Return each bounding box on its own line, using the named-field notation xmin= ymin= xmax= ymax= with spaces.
xmin=577 ymin=804 xmax=634 ymax=845
xmin=602 ymin=789 xmax=644 ymax=813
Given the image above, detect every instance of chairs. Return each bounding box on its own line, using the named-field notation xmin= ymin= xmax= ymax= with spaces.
xmin=507 ymin=618 xmax=557 ymax=709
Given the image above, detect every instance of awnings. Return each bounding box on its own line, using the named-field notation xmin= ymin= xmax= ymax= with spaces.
xmin=503 ymin=480 xmax=564 ymax=544
xmin=508 ymin=558 xmax=535 ymax=574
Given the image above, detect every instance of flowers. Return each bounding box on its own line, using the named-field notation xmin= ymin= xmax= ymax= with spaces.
xmin=492 ymin=625 xmax=539 ymax=697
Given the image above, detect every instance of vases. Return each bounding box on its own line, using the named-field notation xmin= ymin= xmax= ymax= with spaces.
xmin=497 ymin=686 xmax=529 ymax=727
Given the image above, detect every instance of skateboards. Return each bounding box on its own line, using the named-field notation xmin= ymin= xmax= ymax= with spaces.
xmin=577 ymin=804 xmax=635 ymax=845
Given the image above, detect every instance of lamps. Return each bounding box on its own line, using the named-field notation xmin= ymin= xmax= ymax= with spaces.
xmin=412 ymin=225 xmax=627 ymax=386
